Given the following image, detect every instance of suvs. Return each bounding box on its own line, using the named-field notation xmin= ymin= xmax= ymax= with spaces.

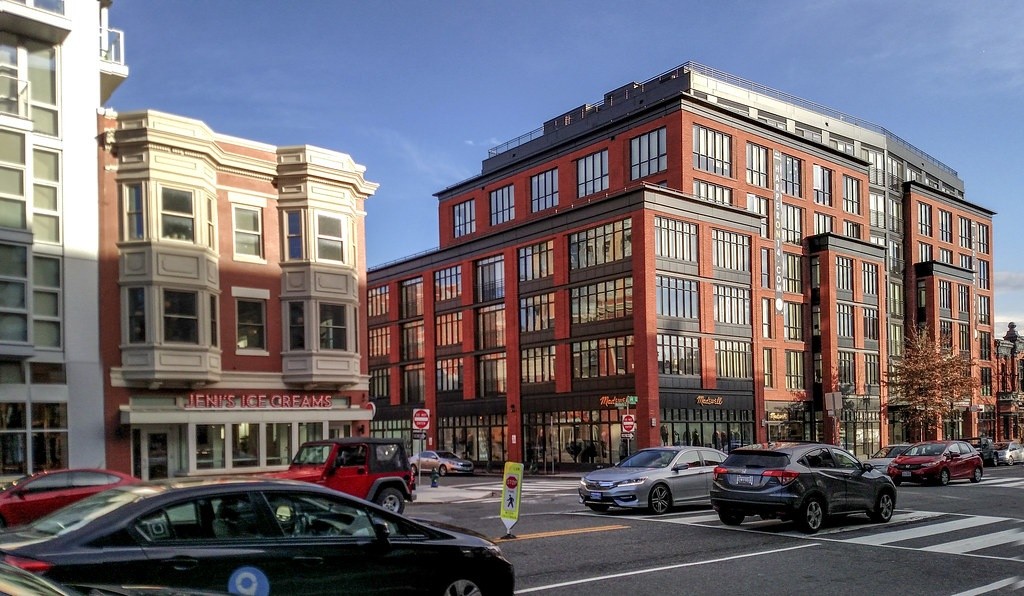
xmin=954 ymin=437 xmax=999 ymax=467
xmin=259 ymin=438 xmax=416 ymax=516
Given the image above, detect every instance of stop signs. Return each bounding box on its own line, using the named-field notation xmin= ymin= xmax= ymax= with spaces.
xmin=623 ymin=416 xmax=634 ymax=431
xmin=414 ymin=410 xmax=429 ymax=428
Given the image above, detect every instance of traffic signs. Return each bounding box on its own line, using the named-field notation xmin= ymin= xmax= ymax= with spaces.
xmin=412 ymin=429 xmax=426 ymax=440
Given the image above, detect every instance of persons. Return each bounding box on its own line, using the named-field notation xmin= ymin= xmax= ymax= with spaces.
xmin=467 ymin=434 xmax=474 ymax=458
xmin=721 ymin=431 xmax=727 ymax=453
xmin=538 ymin=428 xmax=547 ymax=461
xmin=430 ymin=467 xmax=438 ymax=482
xmin=660 ymin=424 xmax=669 ymax=446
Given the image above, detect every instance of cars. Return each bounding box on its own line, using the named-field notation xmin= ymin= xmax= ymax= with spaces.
xmin=710 ymin=442 xmax=898 ymax=535
xmin=578 ymin=446 xmax=729 ymax=514
xmin=0 ymin=469 xmax=144 ymax=528
xmin=408 ymin=450 xmax=474 ymax=477
xmin=992 ymin=441 xmax=1024 ymax=466
xmin=0 ymin=479 xmax=516 ymax=596
xmin=885 ymin=441 xmax=984 ymax=486
xmin=860 ymin=444 xmax=915 ymax=471
